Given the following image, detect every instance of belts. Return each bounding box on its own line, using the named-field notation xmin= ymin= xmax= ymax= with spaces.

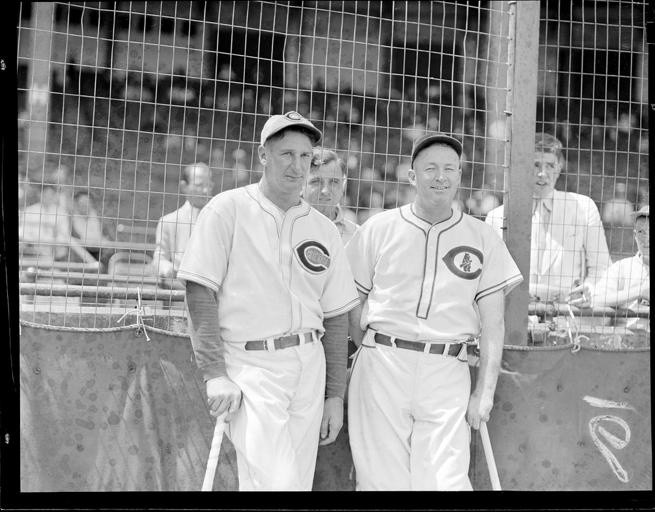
xmin=242 ymin=329 xmax=322 ymax=352
xmin=372 ymin=331 xmax=465 ymax=358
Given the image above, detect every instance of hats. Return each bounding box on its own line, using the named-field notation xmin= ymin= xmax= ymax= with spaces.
xmin=629 ymin=204 xmax=650 ymax=219
xmin=409 ymin=128 xmax=464 ymax=170
xmin=258 ymin=111 xmax=324 ymax=150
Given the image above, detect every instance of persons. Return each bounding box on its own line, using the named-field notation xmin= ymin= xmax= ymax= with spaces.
xmin=18 ymin=180 xmax=73 ymax=265
xmin=150 ymin=162 xmax=218 ymax=280
xmin=344 ymin=134 xmax=525 ymax=490
xmin=66 ymin=191 xmax=117 ymax=270
xmin=300 ymin=147 xmax=365 ymax=241
xmin=484 ymin=131 xmax=615 ymax=330
xmin=589 ymin=204 xmax=652 ymax=334
xmin=172 ymin=108 xmax=366 ymax=492
